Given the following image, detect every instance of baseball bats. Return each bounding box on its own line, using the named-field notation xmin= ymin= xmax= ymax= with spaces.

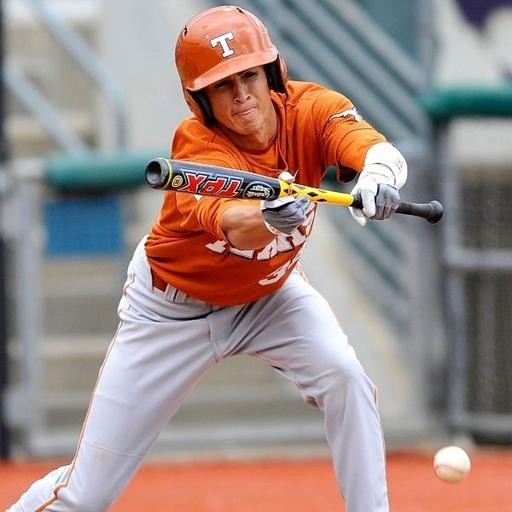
xmin=145 ymin=158 xmax=443 ymax=224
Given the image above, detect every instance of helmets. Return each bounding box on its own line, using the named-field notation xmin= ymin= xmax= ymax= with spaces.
xmin=175 ymin=5 xmax=288 ymax=125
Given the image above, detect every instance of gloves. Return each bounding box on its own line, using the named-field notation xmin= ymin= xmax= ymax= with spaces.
xmin=347 ymin=142 xmax=408 ymax=225
xmin=259 ymin=171 xmax=311 ymax=237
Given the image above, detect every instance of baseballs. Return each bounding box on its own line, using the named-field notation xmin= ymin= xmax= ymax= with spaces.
xmin=434 ymin=446 xmax=470 ymax=482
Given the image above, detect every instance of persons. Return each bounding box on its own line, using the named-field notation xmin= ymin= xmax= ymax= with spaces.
xmin=6 ymin=3 xmax=410 ymax=512
